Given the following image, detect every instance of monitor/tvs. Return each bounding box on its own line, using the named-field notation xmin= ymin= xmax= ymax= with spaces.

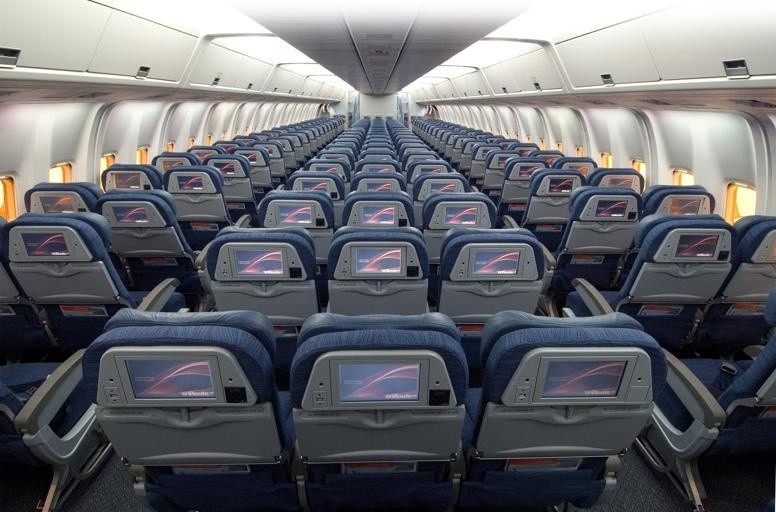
xmin=302 ymin=181 xmax=332 ymax=198
xmin=112 ymin=173 xmax=142 ymax=190
xmin=442 ymin=206 xmax=481 ymax=227
xmin=470 ymin=144 xmax=561 ymax=167
xmin=160 ymin=159 xmax=184 ymax=172
xmin=213 ymin=161 xmax=237 ymax=175
xmin=108 ymin=205 xmax=152 ymax=225
xmin=228 ymin=247 xmax=289 ymax=278
xmin=22 ymin=233 xmax=69 ymax=256
xmin=668 ymin=197 xmax=705 ymax=215
xmin=670 ymin=232 xmax=723 ymax=257
xmin=534 ymin=356 xmax=638 ymax=401
xmin=546 ymin=176 xmax=577 ymax=194
xmin=264 ymin=148 xmax=273 ymax=157
xmin=568 ymin=164 xmax=591 ymax=177
xmin=360 ymin=204 xmax=399 ymax=228
xmin=35 ymin=194 xmax=78 ymax=214
xmin=350 ymin=246 xmax=407 ymax=277
xmin=330 ymin=358 xmax=428 ymax=407
xmin=316 ymin=156 xmax=441 ymax=174
xmin=174 ymin=173 xmax=206 ymax=191
xmin=593 ymin=199 xmax=629 ymax=217
xmin=195 ymin=153 xmax=210 ymax=162
xmin=240 ymin=153 xmax=257 ymax=163
xmin=365 ymin=181 xmax=394 ymax=192
xmin=115 ymin=354 xmax=225 ymax=406
xmin=427 ymin=181 xmax=458 ymax=194
xmin=517 ymin=165 xmax=542 ymax=178
xmin=469 ymin=248 xmax=519 ymax=275
xmin=607 ymin=176 xmax=636 ymax=188
xmin=273 ymin=203 xmax=314 ymax=227
xmin=221 ymin=145 xmax=236 ymax=155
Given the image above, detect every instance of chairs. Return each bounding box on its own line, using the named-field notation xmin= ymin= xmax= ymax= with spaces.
xmin=0 ymin=110 xmax=775 ymax=512
